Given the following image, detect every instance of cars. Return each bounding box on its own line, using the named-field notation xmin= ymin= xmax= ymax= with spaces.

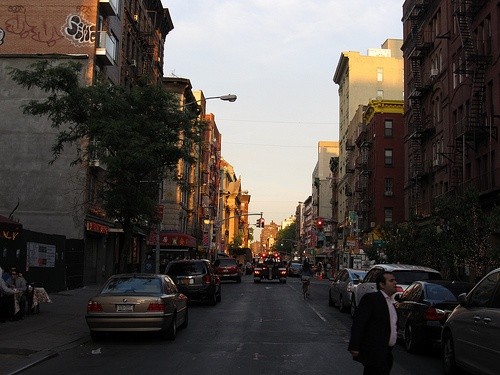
xmin=394 ymin=280 xmax=473 ymax=353
xmin=84 ymin=273 xmax=188 ymax=339
xmin=288 ymin=263 xmax=303 ymax=277
xmin=329 ymin=269 xmax=368 ymax=312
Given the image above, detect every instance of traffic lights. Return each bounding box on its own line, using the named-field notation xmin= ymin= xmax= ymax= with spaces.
xmin=318 ymin=220 xmax=322 ymax=225
xmin=256 ymin=218 xmax=265 ymax=228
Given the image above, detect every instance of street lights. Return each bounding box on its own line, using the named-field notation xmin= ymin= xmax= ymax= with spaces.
xmin=325 ymin=177 xmax=354 ymax=260
xmin=155 ymin=95 xmax=237 ymax=271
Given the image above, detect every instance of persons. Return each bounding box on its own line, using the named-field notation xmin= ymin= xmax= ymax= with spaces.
xmin=245 ymin=260 xmax=251 ymax=276
xmin=314 ymin=262 xmax=322 ymax=274
xmin=164 ymin=255 xmax=172 ymax=268
xmin=300 ymin=258 xmax=312 ymax=294
xmin=0 ymin=266 xmax=29 ymax=321
xmin=324 ymin=263 xmax=328 ymax=277
xmin=348 ymin=271 xmax=401 ymax=375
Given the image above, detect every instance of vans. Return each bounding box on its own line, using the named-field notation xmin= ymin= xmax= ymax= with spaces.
xmin=442 ymin=268 xmax=500 ymax=375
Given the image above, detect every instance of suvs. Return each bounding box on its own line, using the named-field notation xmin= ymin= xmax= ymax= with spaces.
xmin=214 ymin=258 xmax=242 ymax=283
xmin=352 ymin=263 xmax=442 ymax=326
xmin=165 ymin=259 xmax=220 ymax=307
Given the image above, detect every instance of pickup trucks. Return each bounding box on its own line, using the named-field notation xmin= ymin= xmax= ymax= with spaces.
xmin=253 ymin=253 xmax=287 ymax=284
